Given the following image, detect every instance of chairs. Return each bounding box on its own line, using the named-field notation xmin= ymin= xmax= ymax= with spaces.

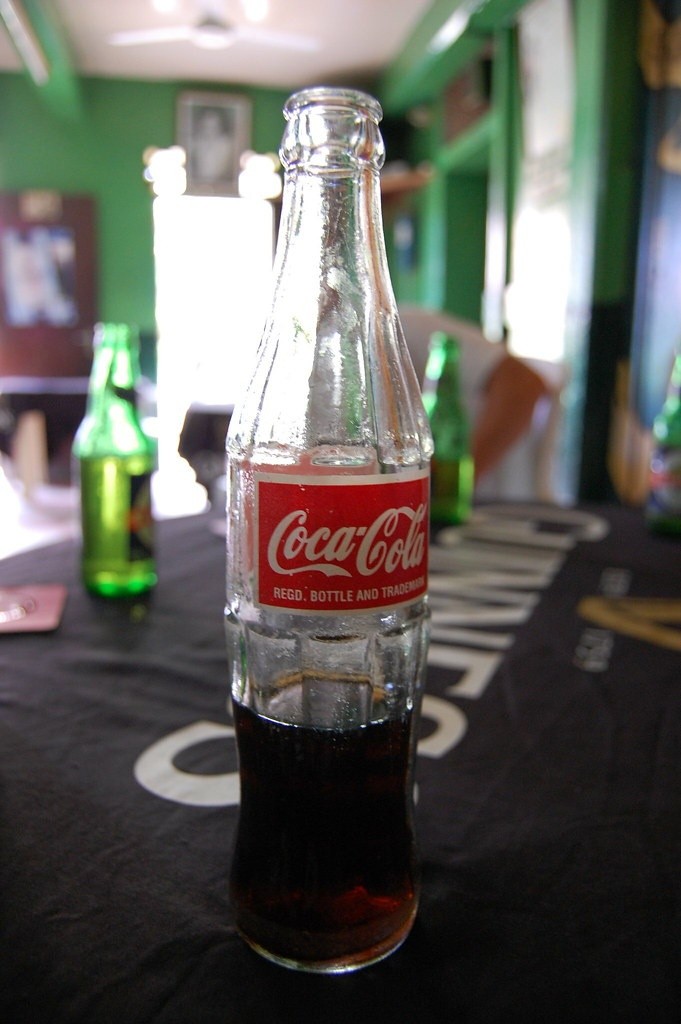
xmin=397 ymin=304 xmax=569 ymax=503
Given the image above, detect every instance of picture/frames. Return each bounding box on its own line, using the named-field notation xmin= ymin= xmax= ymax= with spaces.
xmin=173 ymin=89 xmax=252 ymax=199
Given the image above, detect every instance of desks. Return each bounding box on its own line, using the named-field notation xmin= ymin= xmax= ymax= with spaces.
xmin=0 ymin=495 xmax=681 ymax=1024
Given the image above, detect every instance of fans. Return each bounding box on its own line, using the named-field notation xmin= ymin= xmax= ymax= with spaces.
xmin=107 ymin=0 xmax=316 ymax=54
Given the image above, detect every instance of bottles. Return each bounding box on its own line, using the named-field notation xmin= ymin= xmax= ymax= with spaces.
xmin=644 ymin=355 xmax=681 ymax=538
xmin=72 ymin=323 xmax=159 ymax=595
xmin=420 ymin=331 xmax=475 ymax=527
xmin=219 ymin=89 xmax=433 ymax=979
